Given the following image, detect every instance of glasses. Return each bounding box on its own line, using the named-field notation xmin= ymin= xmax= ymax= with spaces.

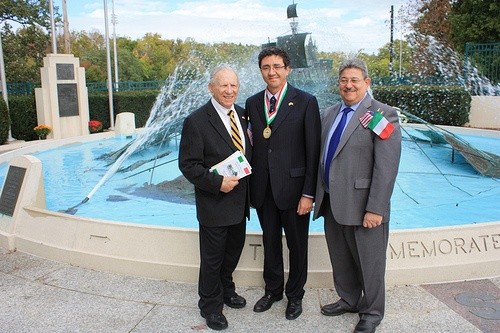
xmin=261 ymin=64 xmax=286 ymax=72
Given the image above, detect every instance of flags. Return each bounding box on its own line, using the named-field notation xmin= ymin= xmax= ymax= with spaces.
xmin=359 ymin=111 xmax=374 ymax=128
xmin=368 ymin=112 xmax=395 ymax=140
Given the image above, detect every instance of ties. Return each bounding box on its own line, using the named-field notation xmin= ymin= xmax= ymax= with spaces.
xmin=324 ymin=108 xmax=353 ymax=192
xmin=228 ymin=111 xmax=244 ymax=154
xmin=269 ymin=96 xmax=276 ymax=113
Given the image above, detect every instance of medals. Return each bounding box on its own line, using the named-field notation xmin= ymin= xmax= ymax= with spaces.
xmin=263 ymin=128 xmax=271 ymax=139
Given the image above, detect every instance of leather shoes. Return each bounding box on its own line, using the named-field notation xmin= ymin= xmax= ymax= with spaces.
xmin=200 ymin=309 xmax=228 ymax=330
xmin=286 ymin=297 xmax=302 ymax=320
xmin=223 ymin=294 xmax=246 ymax=308
xmin=353 ymin=319 xmax=376 ymax=333
xmin=254 ymin=292 xmax=283 ymax=312
xmin=321 ymin=301 xmax=346 ymax=316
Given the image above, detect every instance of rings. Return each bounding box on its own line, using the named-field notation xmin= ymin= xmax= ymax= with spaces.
xmin=307 ymin=211 xmax=309 ymax=213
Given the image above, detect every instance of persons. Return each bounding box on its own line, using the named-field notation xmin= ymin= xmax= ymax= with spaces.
xmin=313 ymin=59 xmax=401 ymax=333
xmin=178 ymin=64 xmax=252 ymax=330
xmin=244 ymin=47 xmax=321 ymax=320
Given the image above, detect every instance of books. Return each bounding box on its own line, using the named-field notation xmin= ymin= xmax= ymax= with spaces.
xmin=209 ymin=150 xmax=252 ymax=181
